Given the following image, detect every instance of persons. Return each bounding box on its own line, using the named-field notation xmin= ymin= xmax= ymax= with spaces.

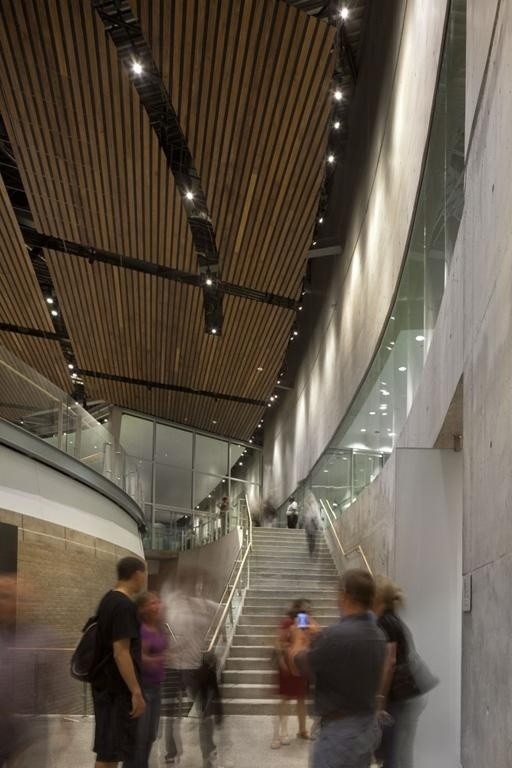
xmin=253 ymin=494 xmax=325 ymax=563
xmin=216 ymin=495 xmax=231 ymax=536
xmin=174 ymin=525 xmax=197 ymax=551
xmin=1 ymin=572 xmax=76 ymax=767
xmin=89 ymin=556 xmax=228 ymax=767
xmin=260 ymin=568 xmax=441 ymax=767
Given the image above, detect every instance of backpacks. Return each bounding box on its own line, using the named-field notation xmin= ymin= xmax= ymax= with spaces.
xmin=71 ymin=594 xmax=126 ymax=682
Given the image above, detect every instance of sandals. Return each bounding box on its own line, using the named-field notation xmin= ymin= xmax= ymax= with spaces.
xmin=297 ymin=731 xmax=317 ymax=741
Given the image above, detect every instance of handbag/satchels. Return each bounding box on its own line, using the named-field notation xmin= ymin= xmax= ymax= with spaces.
xmin=389 ymin=657 xmax=439 ymax=698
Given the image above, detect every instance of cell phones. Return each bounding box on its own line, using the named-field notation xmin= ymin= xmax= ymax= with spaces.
xmin=295 ymin=611 xmax=309 ymax=629
